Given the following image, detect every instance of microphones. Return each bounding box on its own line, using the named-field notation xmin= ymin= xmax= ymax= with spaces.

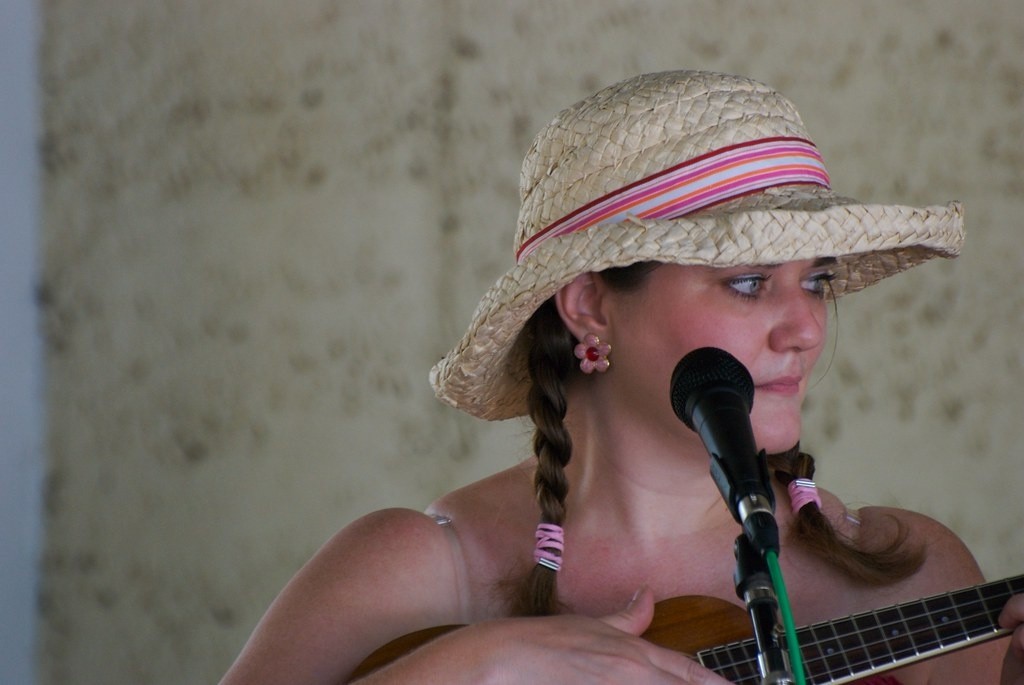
xmin=671 ymin=346 xmax=779 ymax=559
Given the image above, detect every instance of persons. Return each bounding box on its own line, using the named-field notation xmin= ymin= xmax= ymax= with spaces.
xmin=218 ymin=68 xmax=1024 ymax=685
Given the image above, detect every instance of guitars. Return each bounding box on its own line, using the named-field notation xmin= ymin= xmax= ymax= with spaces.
xmin=336 ymin=572 xmax=1024 ymax=684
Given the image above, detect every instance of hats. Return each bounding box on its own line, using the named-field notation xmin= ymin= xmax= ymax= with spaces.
xmin=426 ymin=69 xmax=965 ymax=420
